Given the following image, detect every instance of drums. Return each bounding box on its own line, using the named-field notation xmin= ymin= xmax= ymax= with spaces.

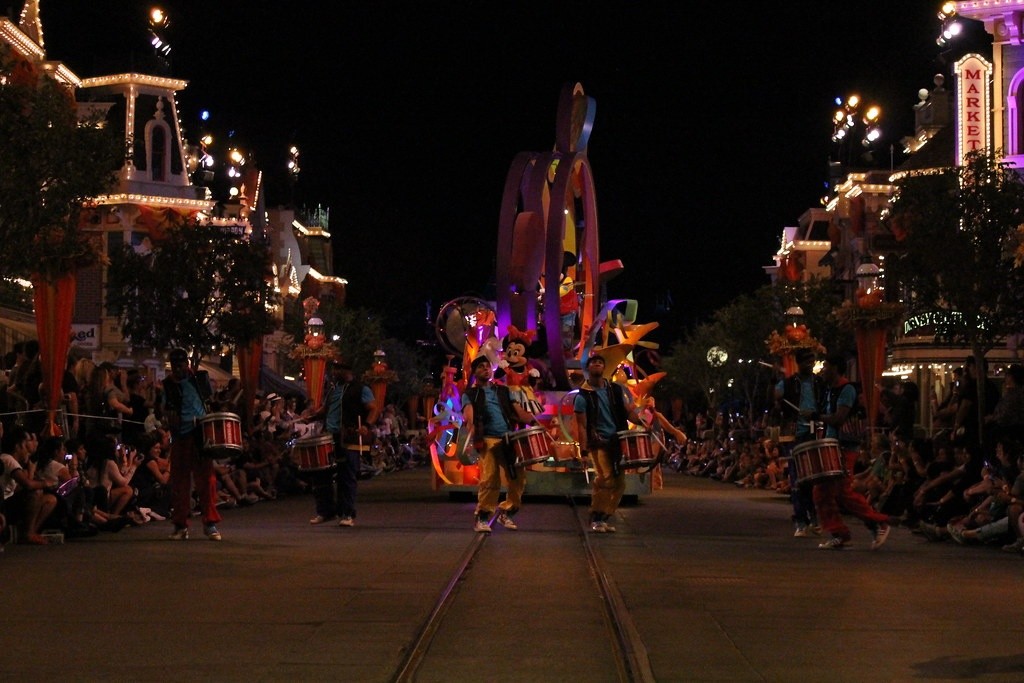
xmin=196 ymin=412 xmax=243 ymax=459
xmin=502 ymin=425 xmax=552 ymax=468
xmin=293 ymin=433 xmax=338 ymax=471
xmin=790 ymin=438 xmax=844 ymax=486
xmin=614 ymin=428 xmax=656 ymax=469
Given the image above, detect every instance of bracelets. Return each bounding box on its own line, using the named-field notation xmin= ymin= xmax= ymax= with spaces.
xmin=530 ymin=420 xmax=537 ymax=426
xmin=581 ymin=450 xmax=588 ymax=457
xmin=811 ymin=412 xmax=820 ymax=420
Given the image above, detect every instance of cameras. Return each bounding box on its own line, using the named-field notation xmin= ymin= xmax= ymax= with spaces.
xmin=65 ymin=455 xmax=72 ymax=461
xmin=137 ymin=453 xmax=144 ymax=460
xmin=116 ymin=444 xmax=121 ymax=450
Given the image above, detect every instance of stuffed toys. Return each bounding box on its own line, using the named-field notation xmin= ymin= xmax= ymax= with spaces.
xmin=494 ymin=325 xmax=540 ymax=432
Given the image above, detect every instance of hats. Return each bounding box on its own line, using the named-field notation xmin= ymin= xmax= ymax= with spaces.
xmin=796 ymin=349 xmax=815 ymax=363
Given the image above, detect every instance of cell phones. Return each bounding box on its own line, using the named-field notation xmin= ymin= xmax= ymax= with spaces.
xmin=984 ymin=461 xmax=989 ymax=471
xmin=895 ymin=440 xmax=899 ymax=446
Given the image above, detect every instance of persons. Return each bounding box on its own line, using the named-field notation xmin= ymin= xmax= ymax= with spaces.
xmin=574 ymin=354 xmax=652 ymax=532
xmin=0 ymin=340 xmax=429 ymax=552
xmin=460 ymin=355 xmax=548 ymax=531
xmin=663 ymin=356 xmax=1024 ymax=553
xmin=798 ymin=354 xmax=891 ymax=549
xmin=153 ymin=349 xmax=229 ymax=542
xmin=781 ymin=347 xmax=826 ymax=537
xmin=302 ymin=358 xmax=379 ymax=526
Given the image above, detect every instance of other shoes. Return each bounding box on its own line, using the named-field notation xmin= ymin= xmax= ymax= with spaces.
xmin=339 ymin=515 xmax=354 ymax=526
xmin=591 ymin=521 xmax=616 ymax=532
xmin=794 ymin=527 xmax=808 ymax=537
xmin=919 ymin=520 xmax=938 ymax=542
xmin=947 ymin=524 xmax=966 ymax=544
xmin=1001 ymin=537 xmax=1024 ymax=552
xmin=203 ymin=524 xmax=221 ymax=541
xmin=473 ymin=521 xmax=492 ymax=532
xmin=871 ymin=523 xmax=892 ymax=550
xmin=495 ymin=513 xmax=518 ymax=530
xmin=818 ymin=537 xmax=843 ymax=549
xmin=810 ymin=523 xmax=823 ymax=535
xmin=309 ymin=514 xmax=324 ymax=524
xmin=168 ymin=527 xmax=189 ymax=540
